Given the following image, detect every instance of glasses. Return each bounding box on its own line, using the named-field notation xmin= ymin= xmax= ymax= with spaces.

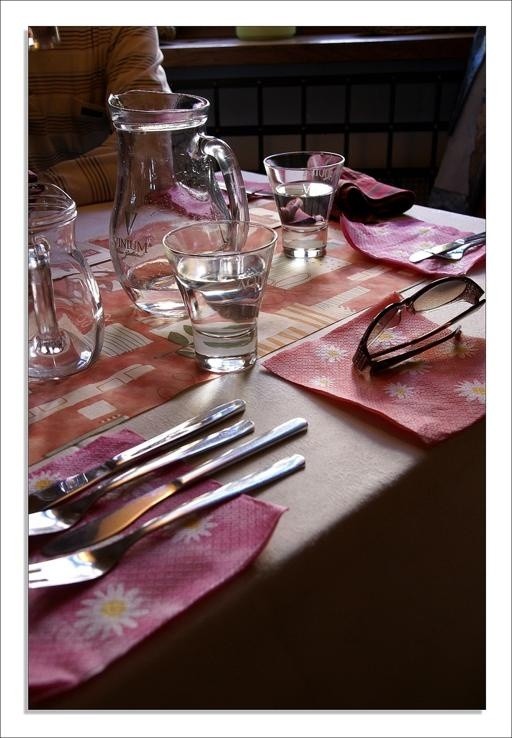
xmin=351 ymin=274 xmax=487 ymax=374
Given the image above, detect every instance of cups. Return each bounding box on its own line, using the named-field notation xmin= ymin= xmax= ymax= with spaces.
xmin=263 ymin=151 xmax=346 ymax=258
xmin=163 ymin=219 xmax=279 ymax=373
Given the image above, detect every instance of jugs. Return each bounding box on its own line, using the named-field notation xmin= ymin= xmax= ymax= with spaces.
xmin=107 ymin=91 xmax=250 ymax=322
xmin=29 ymin=169 xmax=105 ymax=380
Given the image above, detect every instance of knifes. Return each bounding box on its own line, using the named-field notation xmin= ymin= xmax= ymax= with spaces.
xmin=409 ymin=231 xmax=485 ymax=264
xmin=39 ymin=416 xmax=309 ymax=563
xmin=28 ymin=397 xmax=249 ymax=508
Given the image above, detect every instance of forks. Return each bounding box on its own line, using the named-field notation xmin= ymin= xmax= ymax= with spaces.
xmin=29 ymin=454 xmax=336 ymax=605
xmin=426 ymin=239 xmax=485 ymax=270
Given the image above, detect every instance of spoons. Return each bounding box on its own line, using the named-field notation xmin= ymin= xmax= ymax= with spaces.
xmin=28 ymin=418 xmax=261 ymax=535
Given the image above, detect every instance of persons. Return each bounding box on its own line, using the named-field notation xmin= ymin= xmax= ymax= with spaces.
xmin=29 ymin=26 xmax=176 ymax=209
